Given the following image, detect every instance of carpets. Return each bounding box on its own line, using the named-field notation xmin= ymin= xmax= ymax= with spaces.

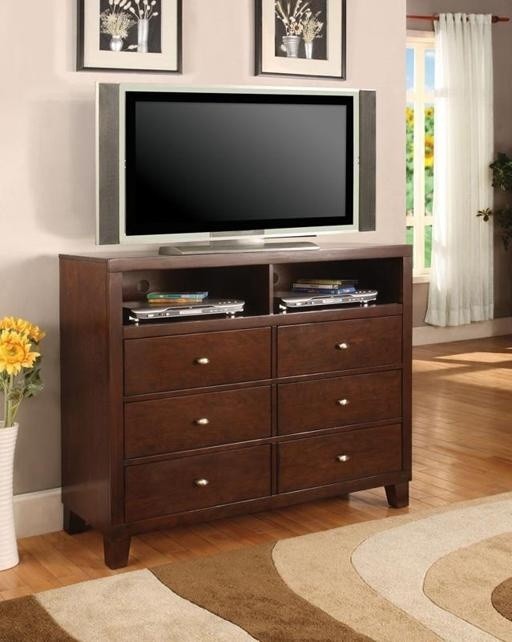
xmin=0 ymin=490 xmax=511 ymax=642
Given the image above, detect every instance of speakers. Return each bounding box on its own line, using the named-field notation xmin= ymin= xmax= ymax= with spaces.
xmin=359 ymin=89 xmax=377 ymax=233
xmin=93 ymin=81 xmax=119 ymax=246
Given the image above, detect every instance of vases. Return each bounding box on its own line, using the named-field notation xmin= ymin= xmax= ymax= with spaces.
xmin=0 ymin=420 xmax=20 ymax=570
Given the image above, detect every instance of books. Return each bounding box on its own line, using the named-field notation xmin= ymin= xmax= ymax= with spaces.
xmin=147 ymin=287 xmax=209 ymax=299
xmin=292 ymin=276 xmax=359 ymax=296
xmin=148 ymin=298 xmax=203 ymax=304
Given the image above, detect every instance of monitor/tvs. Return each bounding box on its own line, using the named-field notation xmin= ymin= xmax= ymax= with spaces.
xmin=118 ymin=83 xmax=359 ymax=254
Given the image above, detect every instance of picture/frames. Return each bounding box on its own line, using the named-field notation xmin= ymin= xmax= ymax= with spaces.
xmin=76 ymin=1 xmax=347 ymax=81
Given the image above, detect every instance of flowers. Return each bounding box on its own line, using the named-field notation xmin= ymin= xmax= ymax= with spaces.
xmin=0 ymin=316 xmax=48 ymax=425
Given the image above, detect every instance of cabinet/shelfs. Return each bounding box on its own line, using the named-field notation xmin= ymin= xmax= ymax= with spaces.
xmin=58 ymin=244 xmax=414 ymax=570
